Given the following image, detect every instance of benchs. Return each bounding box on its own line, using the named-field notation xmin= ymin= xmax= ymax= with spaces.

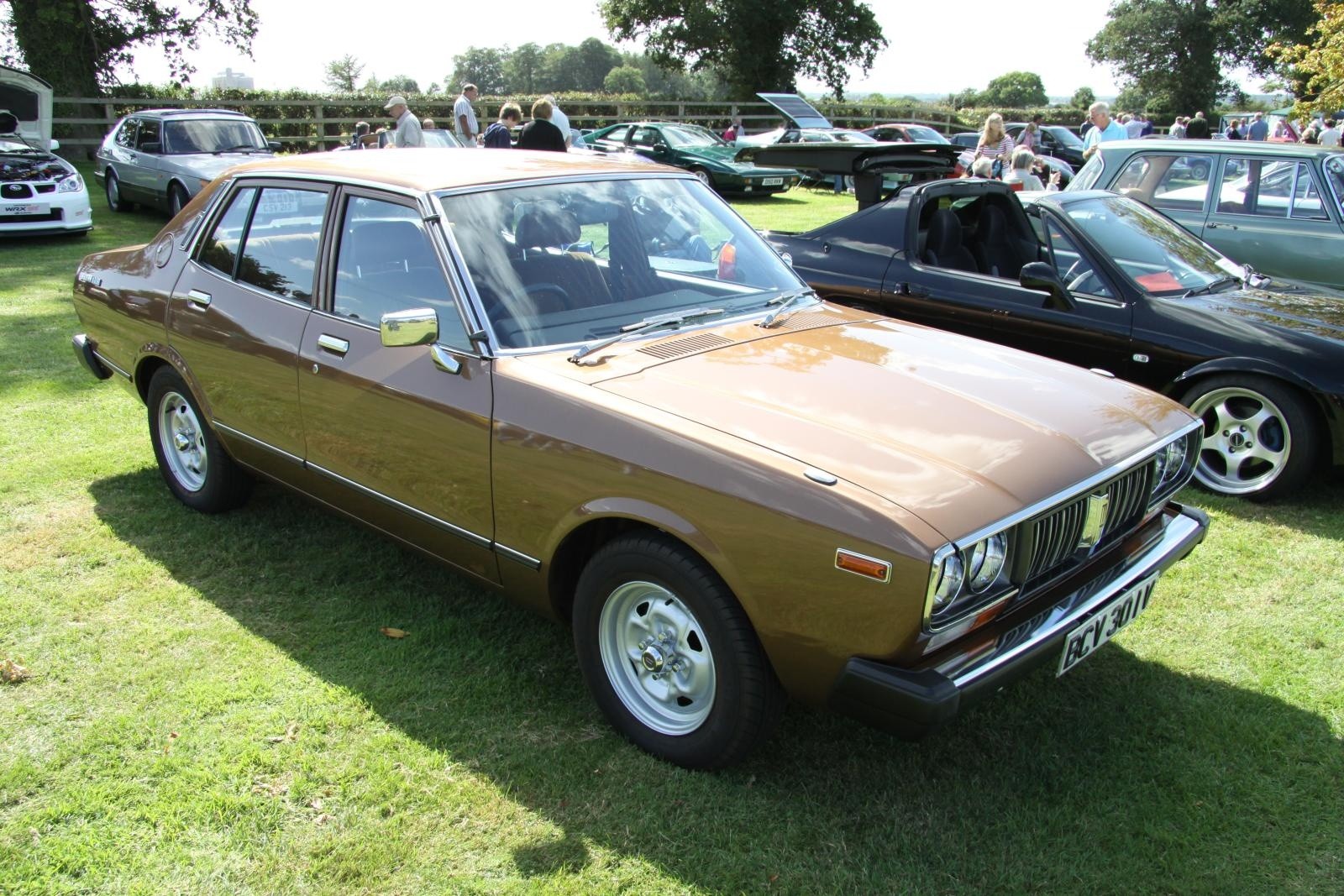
xmin=229 ymin=222 xmax=458 ymax=266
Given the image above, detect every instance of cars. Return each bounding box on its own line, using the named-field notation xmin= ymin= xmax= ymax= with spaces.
xmin=948 ymin=135 xmax=1344 ymax=304
xmin=1 ymin=65 xmax=94 ymax=242
xmin=724 ymin=91 xmax=1344 ymax=205
xmin=568 ymin=121 xmax=800 ymax=199
xmin=71 ymin=147 xmax=1214 ymax=775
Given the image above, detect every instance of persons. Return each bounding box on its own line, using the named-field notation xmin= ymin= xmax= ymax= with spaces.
xmin=1298 ymin=119 xmax=1342 ymax=146
xmin=352 ymin=122 xmax=371 ymax=145
xmin=1169 ymin=111 xmax=1209 ymax=139
xmin=454 ymin=84 xmax=478 ymax=148
xmin=424 ymin=119 xmax=435 ymax=130
xmin=723 ymin=118 xmax=745 ymax=141
xmin=961 ymin=113 xmax=1061 ymax=191
xmin=383 ymin=96 xmax=425 ymax=148
xmin=1226 ymin=112 xmax=1282 ymax=142
xmin=485 ymin=93 xmax=572 ymax=152
xmin=1079 ymin=101 xmax=1153 ymax=160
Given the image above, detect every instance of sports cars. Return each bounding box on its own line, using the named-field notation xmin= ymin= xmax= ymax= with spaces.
xmin=719 ymin=177 xmax=1344 ymax=505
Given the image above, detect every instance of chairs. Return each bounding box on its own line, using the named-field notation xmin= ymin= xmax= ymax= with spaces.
xmin=929 ymin=212 xmax=975 ymax=271
xmin=979 ymin=204 xmax=1023 ymax=271
xmin=498 ymin=214 xmax=613 ymax=306
xmin=346 ymin=221 xmax=438 ymax=315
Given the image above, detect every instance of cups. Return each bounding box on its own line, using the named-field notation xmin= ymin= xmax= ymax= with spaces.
xmin=1052 ymin=168 xmax=1060 ymax=184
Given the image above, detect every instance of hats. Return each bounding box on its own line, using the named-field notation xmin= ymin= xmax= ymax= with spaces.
xmin=732 ymin=124 xmax=739 ymax=130
xmin=384 ymin=96 xmax=406 ymax=110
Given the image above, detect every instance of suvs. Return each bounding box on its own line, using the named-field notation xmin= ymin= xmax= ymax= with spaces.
xmin=94 ymin=107 xmax=284 ymax=222
